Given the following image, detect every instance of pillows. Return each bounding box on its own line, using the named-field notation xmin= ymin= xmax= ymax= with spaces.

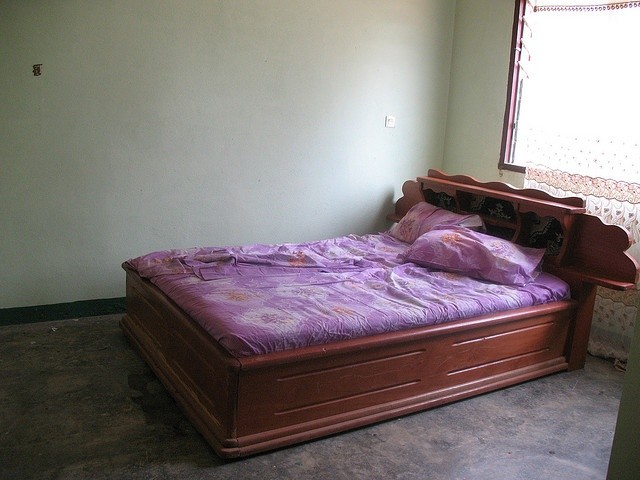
xmin=388 ymin=200 xmax=483 ymax=245
xmin=396 ymin=226 xmax=545 ymax=287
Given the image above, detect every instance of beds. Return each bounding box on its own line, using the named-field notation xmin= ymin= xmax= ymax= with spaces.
xmin=122 ymin=170 xmax=639 ymax=453
